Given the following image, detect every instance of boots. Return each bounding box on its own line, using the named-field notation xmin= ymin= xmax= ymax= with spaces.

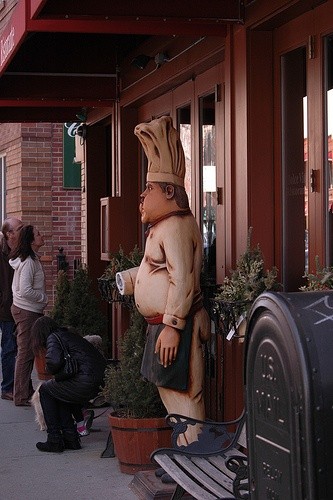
xmin=62 ymin=428 xmax=82 ymax=450
xmin=36 ymin=431 xmax=63 ymax=452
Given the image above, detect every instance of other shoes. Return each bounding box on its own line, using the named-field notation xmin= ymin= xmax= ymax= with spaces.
xmin=2 ymin=393 xmax=13 ymax=400
xmin=20 ymin=401 xmax=31 ymax=406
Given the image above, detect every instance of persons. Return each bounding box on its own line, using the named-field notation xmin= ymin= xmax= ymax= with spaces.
xmin=31 ymin=317 xmax=107 ymax=452
xmin=134 ymin=114 xmax=209 ymax=483
xmin=9 ymin=225 xmax=49 ymax=407
xmin=0 ymin=218 xmax=24 ymax=398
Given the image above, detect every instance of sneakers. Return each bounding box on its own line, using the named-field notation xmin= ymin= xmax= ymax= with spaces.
xmin=83 ymin=406 xmax=94 ymax=428
xmin=76 ymin=426 xmax=90 ymax=435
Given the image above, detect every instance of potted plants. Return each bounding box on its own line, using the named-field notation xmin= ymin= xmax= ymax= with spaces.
xmin=96 ymin=307 xmax=173 ymax=475
xmin=34 ymin=267 xmax=103 ymax=380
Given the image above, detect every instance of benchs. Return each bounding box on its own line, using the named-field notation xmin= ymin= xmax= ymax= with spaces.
xmin=150 ymin=408 xmax=251 ymax=500
